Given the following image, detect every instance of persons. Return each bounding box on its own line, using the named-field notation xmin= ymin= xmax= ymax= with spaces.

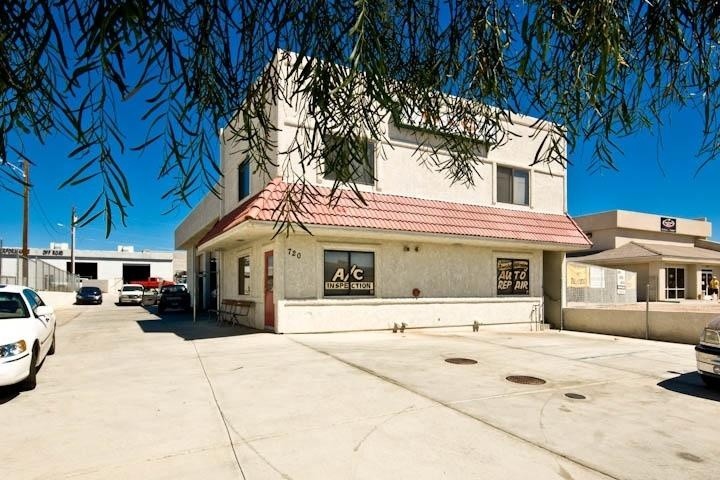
xmin=710 ymin=275 xmax=720 ymax=300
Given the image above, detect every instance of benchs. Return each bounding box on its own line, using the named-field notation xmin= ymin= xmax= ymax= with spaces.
xmin=207 ymin=298 xmax=255 ymax=329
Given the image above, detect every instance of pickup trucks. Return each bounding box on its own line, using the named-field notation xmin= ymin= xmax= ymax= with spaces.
xmin=131 ymin=277 xmax=174 ymax=288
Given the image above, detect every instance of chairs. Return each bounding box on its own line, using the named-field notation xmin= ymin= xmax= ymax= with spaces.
xmin=0 ymin=300 xmax=18 ymax=311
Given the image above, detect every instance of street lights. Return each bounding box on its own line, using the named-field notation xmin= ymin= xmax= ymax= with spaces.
xmin=55 ymin=207 xmax=77 ymax=274
xmin=0 ymin=158 xmax=30 ymax=284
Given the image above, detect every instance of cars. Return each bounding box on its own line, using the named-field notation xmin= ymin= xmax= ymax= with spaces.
xmin=0 ymin=284 xmax=56 ymax=391
xmin=695 ymin=316 xmax=720 ymax=385
xmin=118 ymin=284 xmax=145 ymax=304
xmin=157 ymin=284 xmax=190 ymax=313
xmin=76 ymin=286 xmax=102 ymax=305
